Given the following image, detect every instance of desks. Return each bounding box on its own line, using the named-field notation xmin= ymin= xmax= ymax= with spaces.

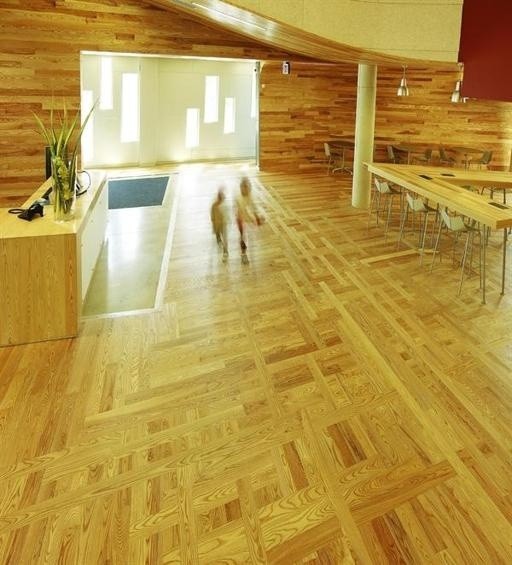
xmin=365 ymin=163 xmax=511 ymax=305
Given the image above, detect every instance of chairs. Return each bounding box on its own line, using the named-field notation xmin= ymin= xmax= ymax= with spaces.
xmin=365 ymin=178 xmax=482 ymax=295
xmin=324 ymin=140 xmax=492 ymax=176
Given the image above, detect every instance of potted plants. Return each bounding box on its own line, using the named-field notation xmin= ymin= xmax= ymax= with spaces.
xmin=26 ymin=88 xmax=103 ymax=221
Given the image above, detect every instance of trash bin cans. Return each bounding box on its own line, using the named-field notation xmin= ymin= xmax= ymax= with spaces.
xmin=45 ymin=145 xmax=68 ymax=180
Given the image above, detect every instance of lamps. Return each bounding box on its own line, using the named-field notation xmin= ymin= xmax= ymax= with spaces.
xmin=397 ymin=64 xmax=409 ymax=96
xmin=449 ymin=63 xmax=465 ymax=105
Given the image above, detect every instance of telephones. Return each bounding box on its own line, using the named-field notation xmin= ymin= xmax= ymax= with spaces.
xmin=18 ymin=187 xmax=52 ymax=220
